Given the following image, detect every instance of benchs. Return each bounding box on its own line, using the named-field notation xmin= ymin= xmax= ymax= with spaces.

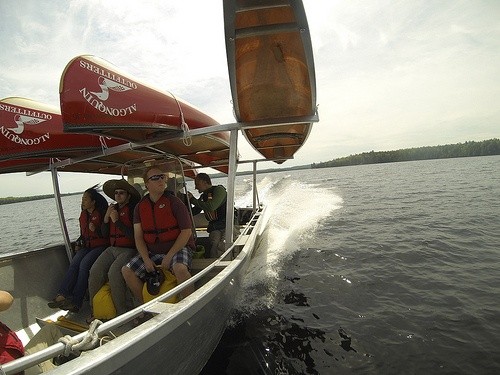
xmin=193 ymin=227 xmax=239 ymax=258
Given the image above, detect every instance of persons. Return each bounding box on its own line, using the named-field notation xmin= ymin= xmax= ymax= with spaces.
xmin=47 ymin=187 xmax=109 ymax=313
xmin=163 ymin=175 xmax=190 ymax=208
xmin=186 ymin=172 xmax=239 ymax=260
xmin=121 ymin=164 xmax=195 ymax=328
xmin=85 ymin=179 xmax=142 ymax=323
xmin=0 ymin=290 xmax=91 ymax=375
xmin=134 ymin=182 xmax=148 ymax=200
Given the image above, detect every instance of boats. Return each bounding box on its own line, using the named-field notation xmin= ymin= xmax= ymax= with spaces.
xmin=0 ymin=0 xmax=321 ymax=375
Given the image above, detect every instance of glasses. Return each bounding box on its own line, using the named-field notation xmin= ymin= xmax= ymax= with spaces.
xmin=113 ymin=190 xmax=128 ymax=195
xmin=145 ymin=173 xmax=168 ymax=183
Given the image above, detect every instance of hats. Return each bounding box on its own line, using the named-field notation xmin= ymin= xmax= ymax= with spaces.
xmin=144 ymin=267 xmax=165 ymax=295
xmin=131 ymin=182 xmax=148 ymax=198
xmin=103 ymin=178 xmax=141 ymax=205
xmin=164 ymin=177 xmax=188 ymax=192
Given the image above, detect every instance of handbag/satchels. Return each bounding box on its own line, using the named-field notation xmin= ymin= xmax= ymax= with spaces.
xmin=203 ymin=193 xmax=218 ymax=222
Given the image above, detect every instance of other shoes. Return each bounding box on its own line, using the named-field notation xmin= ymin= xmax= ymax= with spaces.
xmin=48 ymin=298 xmax=70 ymax=308
xmin=61 ymin=305 xmax=79 ymax=314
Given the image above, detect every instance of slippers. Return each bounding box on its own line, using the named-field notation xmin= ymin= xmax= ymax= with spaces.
xmin=130 ymin=316 xmax=145 ymax=327
xmin=85 ymin=311 xmax=97 ymax=324
